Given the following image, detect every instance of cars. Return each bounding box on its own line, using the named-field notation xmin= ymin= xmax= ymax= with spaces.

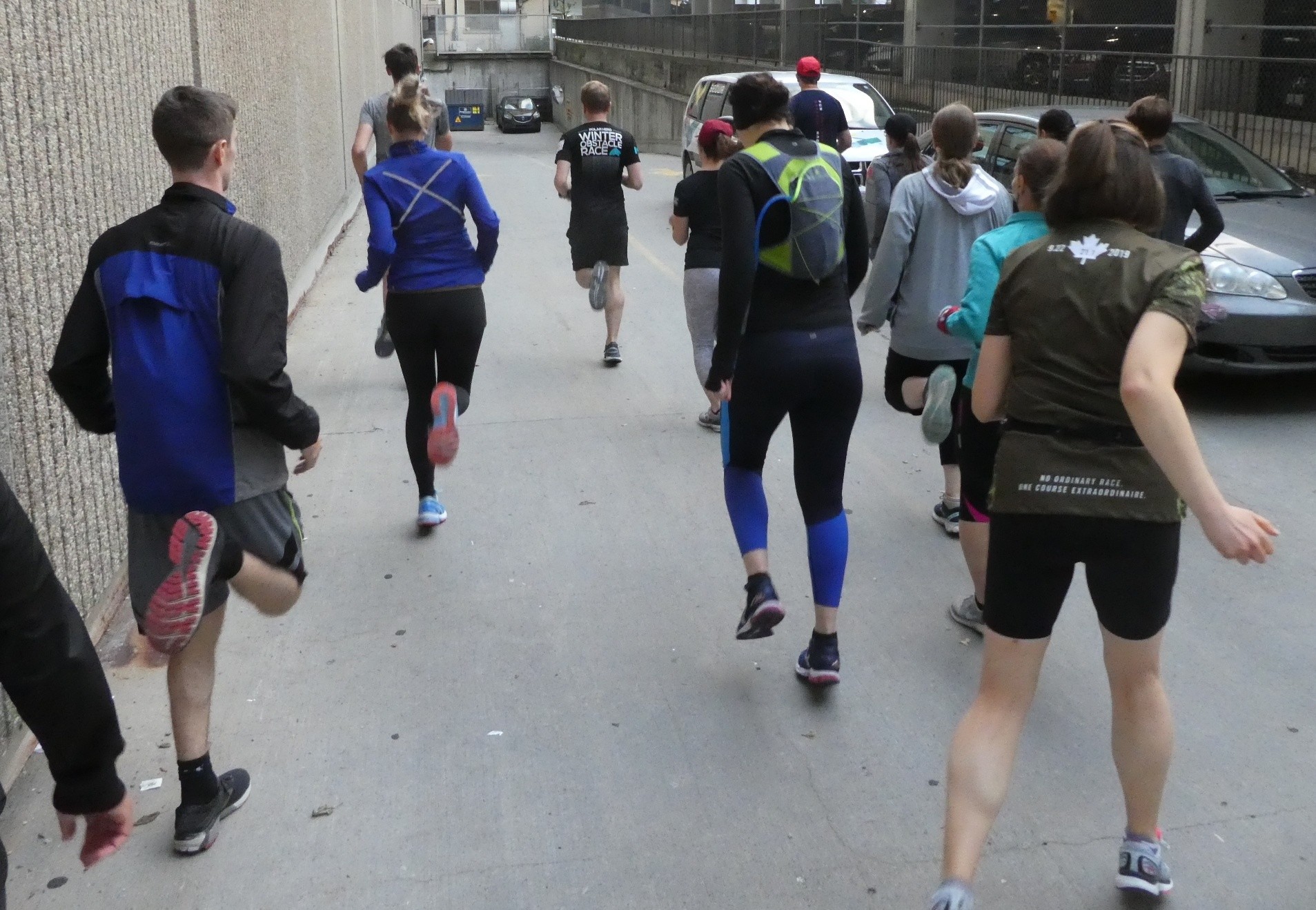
xmin=917 ymin=107 xmax=1316 ymax=403
xmin=496 ymin=96 xmax=541 ymax=134
xmin=765 ymin=24 xmax=1174 ymax=102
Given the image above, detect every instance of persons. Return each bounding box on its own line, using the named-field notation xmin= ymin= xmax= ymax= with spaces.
xmin=937 ymin=138 xmax=1065 ymax=634
xmin=350 ymin=43 xmax=453 ymax=358
xmin=0 ymin=471 xmax=130 ymax=909
xmin=1125 ymin=95 xmax=1224 ymax=253
xmin=554 ymin=80 xmax=643 ymax=362
xmin=670 ymin=119 xmax=744 ymax=433
xmin=47 ymin=86 xmax=324 ymax=854
xmin=865 ymin=112 xmax=937 ymax=260
xmin=927 ymin=118 xmax=1280 ymax=910
xmin=703 ymin=71 xmax=870 ymax=683
xmin=787 ymin=56 xmax=851 ymax=153
xmin=354 ymin=71 xmax=501 ymax=529
xmin=856 ymin=101 xmax=1014 ymax=534
xmin=1013 ymin=108 xmax=1075 ymax=214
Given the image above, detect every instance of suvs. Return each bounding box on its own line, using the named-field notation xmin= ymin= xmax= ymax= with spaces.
xmin=681 ymin=71 xmax=896 ymax=203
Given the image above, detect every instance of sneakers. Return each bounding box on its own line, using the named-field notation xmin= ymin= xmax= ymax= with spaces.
xmin=931 ymin=491 xmax=961 ymax=533
xmin=698 ymin=406 xmax=721 ymax=432
xmin=374 ymin=312 xmax=395 ymax=358
xmin=795 ymin=639 xmax=840 ymax=684
xmin=603 ymin=341 xmax=622 ymax=363
xmin=142 ymin=510 xmax=228 ymax=655
xmin=950 ymin=592 xmax=985 ymax=636
xmin=426 ymin=381 xmax=460 ymax=464
xmin=736 ymin=581 xmax=784 ymax=641
xmin=589 ymin=259 xmax=610 ymax=310
xmin=416 ymin=490 xmax=447 ymax=526
xmin=171 ymin=766 xmax=253 ymax=854
xmin=1116 ymin=828 xmax=1173 ymax=895
xmin=920 ymin=364 xmax=957 ymax=444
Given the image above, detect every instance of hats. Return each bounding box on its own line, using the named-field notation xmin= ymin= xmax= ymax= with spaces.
xmin=796 ymin=55 xmax=820 ymax=78
xmin=694 ymin=119 xmax=733 ymax=146
